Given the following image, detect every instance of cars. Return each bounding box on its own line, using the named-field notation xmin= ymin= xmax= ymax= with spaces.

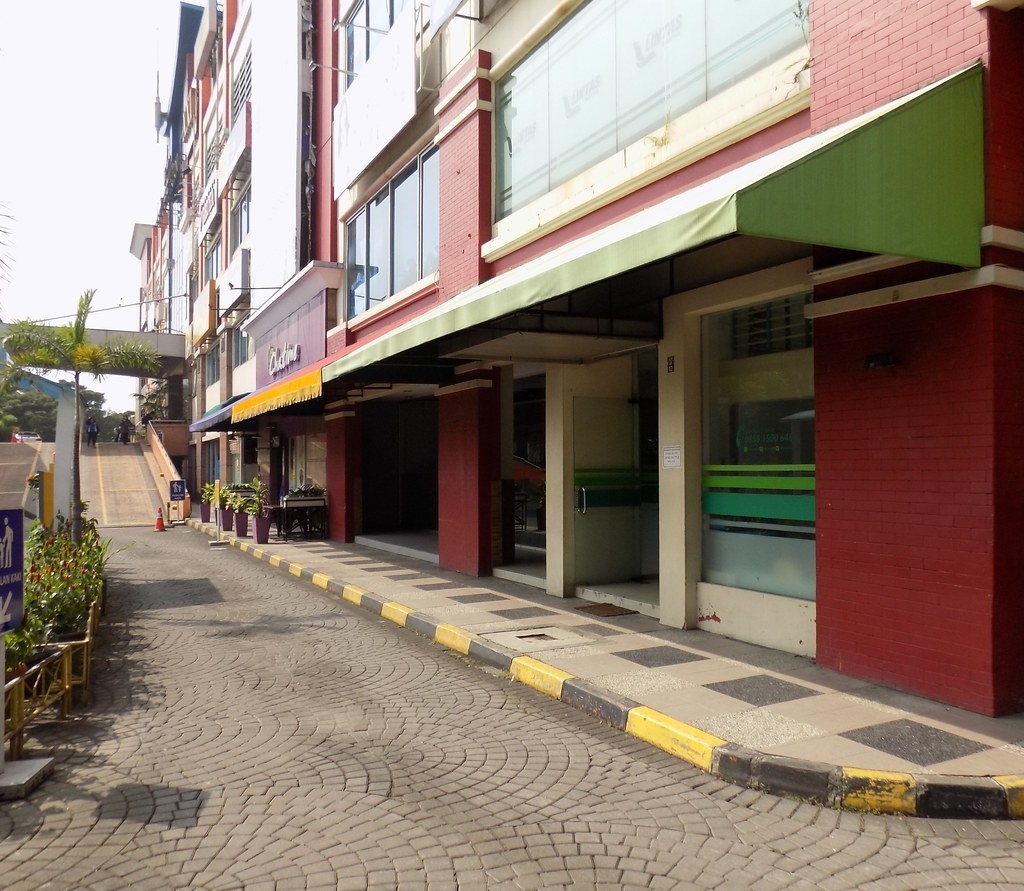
xmin=14 ymin=430 xmax=42 ymax=442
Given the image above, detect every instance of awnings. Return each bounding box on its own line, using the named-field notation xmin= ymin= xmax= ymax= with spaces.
xmin=189 ymin=314 xmax=421 ymax=432
xmin=322 ymin=60 xmax=985 ymax=386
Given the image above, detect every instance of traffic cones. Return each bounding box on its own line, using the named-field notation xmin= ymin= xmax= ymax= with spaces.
xmin=153 ymin=507 xmax=167 ymax=532
xmin=10 ymin=430 xmax=16 ymax=442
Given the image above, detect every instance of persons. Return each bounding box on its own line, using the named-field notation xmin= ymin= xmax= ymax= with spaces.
xmin=86 ymin=417 xmax=99 ymax=448
xmin=118 ymin=414 xmax=133 ymax=445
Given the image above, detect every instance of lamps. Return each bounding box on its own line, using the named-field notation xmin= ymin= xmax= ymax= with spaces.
xmin=863 ymin=353 xmax=901 ymax=367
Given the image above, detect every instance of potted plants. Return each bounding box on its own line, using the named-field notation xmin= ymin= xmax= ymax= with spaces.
xmin=231 ymin=493 xmax=253 ymax=538
xmin=250 ymin=479 xmax=270 ymax=545
xmin=219 ymin=485 xmax=238 ymax=532
xmin=198 ymin=484 xmax=216 ymax=524
